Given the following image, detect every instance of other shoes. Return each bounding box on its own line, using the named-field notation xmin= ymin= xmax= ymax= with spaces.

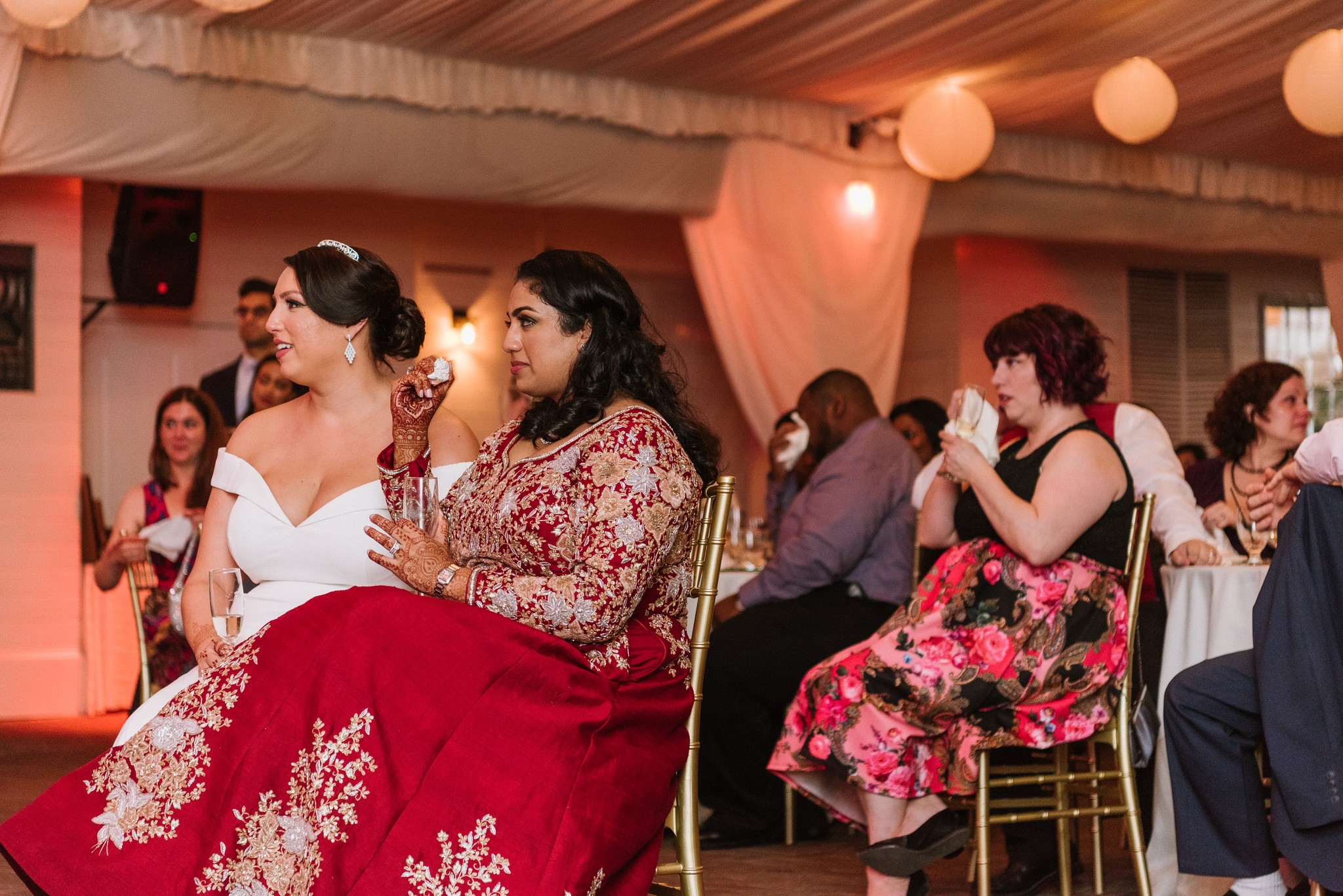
xmin=858 ymin=812 xmax=968 ymax=878
xmin=903 ymin=872 xmax=933 ymax=896
xmin=697 ymin=816 xmax=781 ymax=845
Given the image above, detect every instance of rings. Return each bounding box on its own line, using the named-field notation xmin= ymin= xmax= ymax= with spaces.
xmin=407 ymin=366 xmax=413 ymax=375
xmin=1220 ymin=513 xmax=1225 ymax=519
xmin=389 ymin=540 xmax=400 ymax=554
xmin=1209 ymin=552 xmax=1216 ymax=557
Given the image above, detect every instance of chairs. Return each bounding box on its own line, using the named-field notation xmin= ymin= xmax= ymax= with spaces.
xmin=648 ymin=473 xmax=1155 ymax=896
xmin=120 ymin=529 xmax=161 ymax=705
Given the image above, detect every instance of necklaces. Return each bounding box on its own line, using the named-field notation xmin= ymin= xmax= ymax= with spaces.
xmin=1231 ymin=452 xmax=1290 ymax=497
xmin=540 ymin=434 xmax=552 ymax=445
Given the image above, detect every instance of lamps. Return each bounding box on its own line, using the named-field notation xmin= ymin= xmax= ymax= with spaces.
xmin=848 ymin=80 xmax=994 ymax=181
xmin=1093 ymin=55 xmax=1178 ymax=144
xmin=1282 ymin=29 xmax=1343 ymax=138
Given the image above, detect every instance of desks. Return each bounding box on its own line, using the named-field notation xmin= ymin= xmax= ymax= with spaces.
xmin=1145 ymin=563 xmax=1270 ymax=896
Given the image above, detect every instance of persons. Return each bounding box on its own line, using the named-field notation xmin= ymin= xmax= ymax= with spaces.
xmin=1163 ymin=418 xmax=1343 ymax=896
xmin=250 ymin=353 xmax=301 ymax=414
xmin=110 ymin=241 xmax=482 ymax=745
xmin=1176 ymin=444 xmax=1206 ymax=471
xmin=766 ymin=303 xmax=1222 ymax=896
xmin=1182 ymin=361 xmax=1313 ymax=563
xmin=196 ymin=278 xmax=310 ymax=429
xmin=0 ymin=248 xmax=722 ymax=896
xmin=695 ymin=366 xmax=948 ymax=849
xmin=94 ymin=385 xmax=228 ymax=715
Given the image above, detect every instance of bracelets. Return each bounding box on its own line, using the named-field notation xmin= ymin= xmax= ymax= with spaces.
xmin=936 ymin=471 xmax=962 ymax=484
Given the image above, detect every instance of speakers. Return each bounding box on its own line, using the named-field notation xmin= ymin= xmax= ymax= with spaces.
xmin=108 ymin=186 xmax=203 ymax=308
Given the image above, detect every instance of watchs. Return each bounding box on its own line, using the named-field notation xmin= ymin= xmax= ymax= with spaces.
xmin=735 ymin=595 xmax=745 ymax=614
xmin=433 ymin=564 xmax=462 ymax=600
